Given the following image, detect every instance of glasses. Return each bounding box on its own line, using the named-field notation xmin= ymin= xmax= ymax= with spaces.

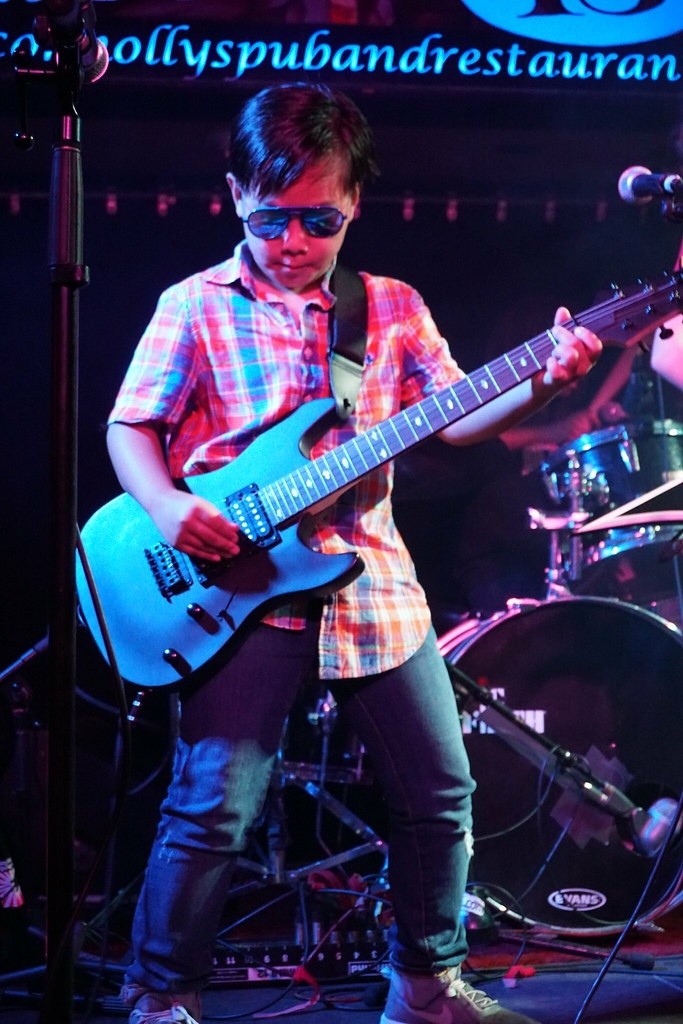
xmin=241 ymin=189 xmax=353 ymax=239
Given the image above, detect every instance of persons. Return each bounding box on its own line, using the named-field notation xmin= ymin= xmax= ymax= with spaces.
xmin=499 ymin=371 xmax=638 ymax=602
xmin=105 ymin=84 xmax=602 ymax=1024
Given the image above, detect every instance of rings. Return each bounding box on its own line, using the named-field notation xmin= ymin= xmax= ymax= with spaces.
xmin=609 ymin=407 xmax=616 ymax=415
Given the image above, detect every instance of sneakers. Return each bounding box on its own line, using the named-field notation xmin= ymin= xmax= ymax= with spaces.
xmin=380 ymin=964 xmax=540 ymax=1024
xmin=129 ymin=1004 xmax=200 ymax=1024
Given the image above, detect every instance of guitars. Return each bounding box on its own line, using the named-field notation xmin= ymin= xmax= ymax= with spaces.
xmin=71 ymin=269 xmax=683 ymax=700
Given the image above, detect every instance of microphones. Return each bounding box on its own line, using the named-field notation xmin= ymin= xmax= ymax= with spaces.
xmin=618 ymin=165 xmax=682 ymax=206
xmin=44 ymin=0 xmax=109 ymax=84
xmin=632 ymin=797 xmax=683 ymax=857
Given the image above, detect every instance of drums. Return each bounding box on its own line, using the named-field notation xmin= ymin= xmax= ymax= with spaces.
xmin=434 ymin=593 xmax=683 ymax=937
xmin=525 ymin=418 xmax=683 ymax=607
